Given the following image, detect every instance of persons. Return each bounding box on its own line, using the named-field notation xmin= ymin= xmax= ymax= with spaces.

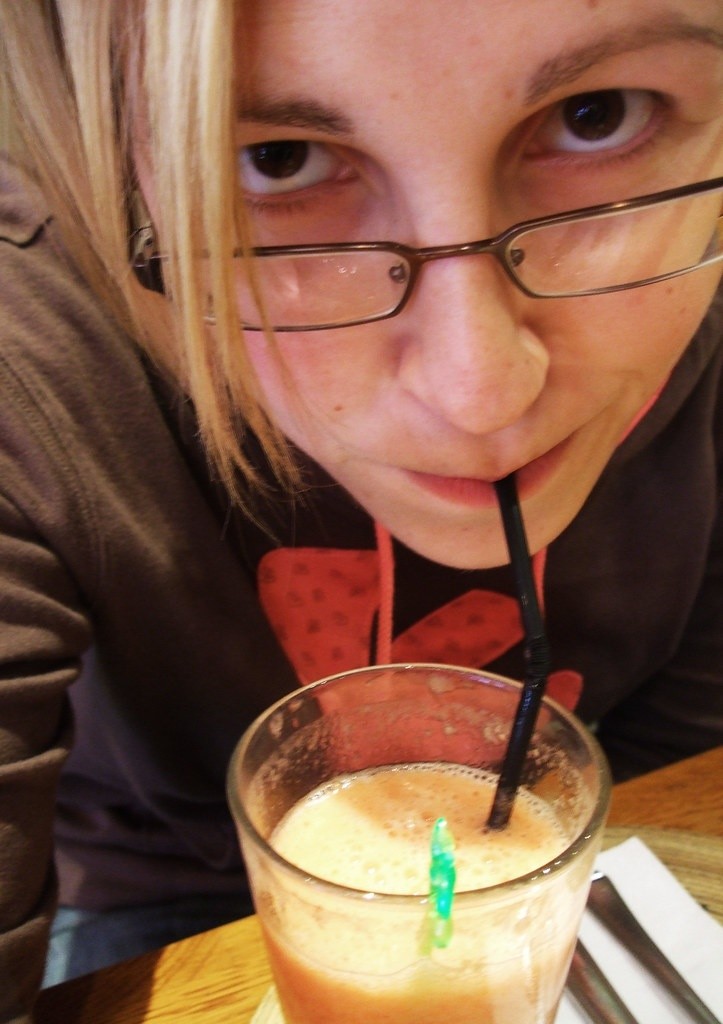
xmin=0 ymin=0 xmax=723 ymax=1024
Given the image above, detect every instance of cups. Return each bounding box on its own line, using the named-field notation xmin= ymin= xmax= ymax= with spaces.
xmin=227 ymin=663 xmax=608 ymax=1024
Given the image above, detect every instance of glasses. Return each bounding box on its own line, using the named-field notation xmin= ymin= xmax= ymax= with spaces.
xmin=123 ymin=175 xmax=723 ymax=332
